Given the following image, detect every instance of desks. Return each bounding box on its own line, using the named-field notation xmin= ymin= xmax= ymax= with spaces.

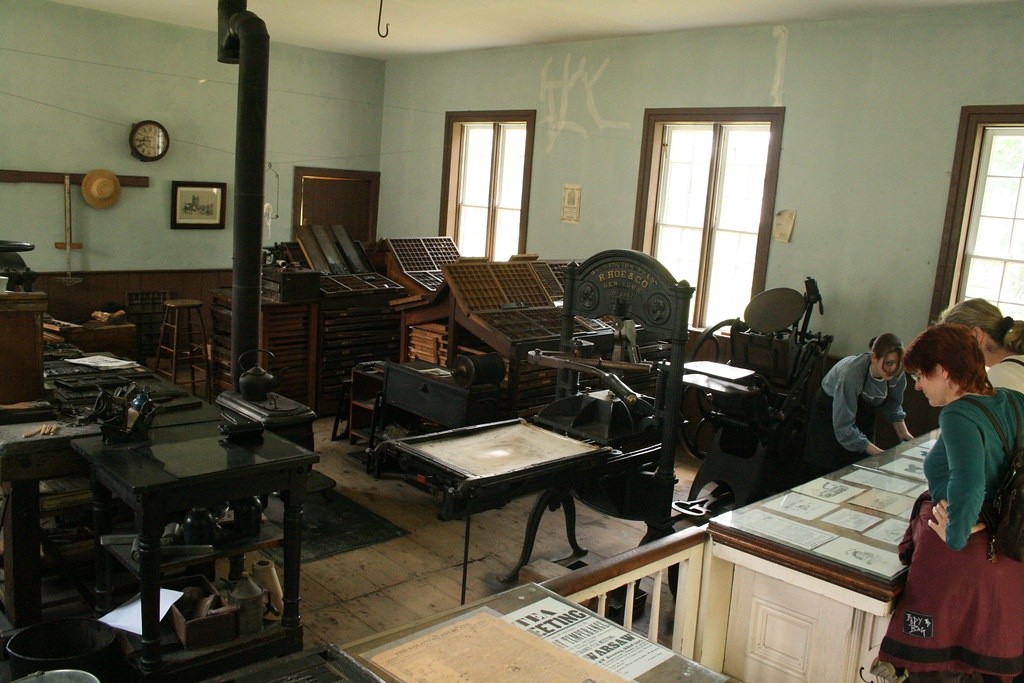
xmin=336 ymin=581 xmax=731 ymax=683
xmin=43 ymin=351 xmax=321 ymax=683
xmin=697 ymin=427 xmax=941 ymax=683
xmin=368 ymin=360 xmax=510 ymax=522
xmin=209 ymin=288 xmax=408 ymax=420
xmin=0 ymin=289 xmax=48 ymax=407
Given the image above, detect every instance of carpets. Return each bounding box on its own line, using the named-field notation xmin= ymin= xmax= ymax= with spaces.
xmin=257 ymin=488 xmax=412 ymax=571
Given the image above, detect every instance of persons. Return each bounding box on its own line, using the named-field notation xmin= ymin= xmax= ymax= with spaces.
xmin=937 ymin=298 xmax=1024 ymax=393
xmin=804 ymin=333 xmax=914 ymax=481
xmin=878 ymin=323 xmax=1024 ymax=682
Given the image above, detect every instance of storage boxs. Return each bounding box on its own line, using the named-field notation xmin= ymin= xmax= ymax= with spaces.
xmin=161 ymin=574 xmax=239 ymax=651
xmin=62 ymin=321 xmax=138 ymax=359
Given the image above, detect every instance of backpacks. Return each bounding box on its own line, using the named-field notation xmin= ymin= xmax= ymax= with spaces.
xmin=957 ymin=393 xmax=1024 ymax=564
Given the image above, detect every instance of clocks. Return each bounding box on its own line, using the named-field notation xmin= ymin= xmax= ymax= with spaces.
xmin=129 ymin=120 xmax=170 ymax=162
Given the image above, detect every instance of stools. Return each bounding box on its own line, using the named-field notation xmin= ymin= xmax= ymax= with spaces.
xmin=153 ymin=299 xmax=212 ymax=391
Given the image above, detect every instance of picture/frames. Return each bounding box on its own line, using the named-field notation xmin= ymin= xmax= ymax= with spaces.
xmin=171 ymin=180 xmax=226 ymax=230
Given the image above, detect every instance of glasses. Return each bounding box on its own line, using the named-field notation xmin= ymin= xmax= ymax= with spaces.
xmin=911 ymin=371 xmax=923 ymax=381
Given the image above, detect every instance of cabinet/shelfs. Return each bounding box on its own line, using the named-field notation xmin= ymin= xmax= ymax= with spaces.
xmin=349 ymin=366 xmax=384 ymax=444
